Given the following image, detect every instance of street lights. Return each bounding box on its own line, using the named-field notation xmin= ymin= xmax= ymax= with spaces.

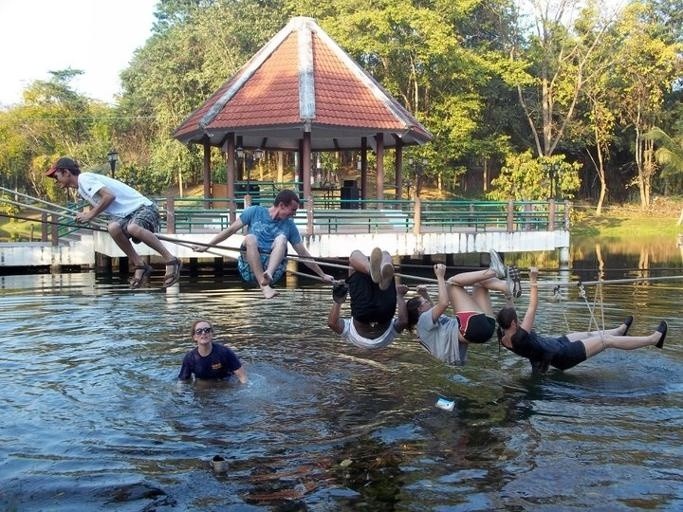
xmin=544 ymin=162 xmax=559 ymax=201
xmin=356 ymin=161 xmax=361 ymax=178
xmin=106 ymin=148 xmax=119 ymax=178
xmin=235 ymin=145 xmax=263 ymax=195
xmin=407 ymin=155 xmax=428 ymax=201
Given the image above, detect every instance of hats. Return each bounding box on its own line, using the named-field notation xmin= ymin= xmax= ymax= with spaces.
xmin=44 ymin=157 xmax=75 ymax=177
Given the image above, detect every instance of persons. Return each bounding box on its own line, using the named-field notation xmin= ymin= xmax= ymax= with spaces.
xmin=178 ymin=320 xmax=247 ymax=390
xmin=46 ymin=158 xmax=183 ymax=290
xmin=407 ymin=248 xmax=522 ymax=366
xmin=494 ymin=268 xmax=665 ymax=370
xmin=195 ymin=189 xmax=334 ymax=300
xmin=329 ymin=248 xmax=409 ymax=351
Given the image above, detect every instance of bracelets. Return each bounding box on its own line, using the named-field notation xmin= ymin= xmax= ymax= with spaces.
xmin=530 ymin=281 xmax=538 ymax=287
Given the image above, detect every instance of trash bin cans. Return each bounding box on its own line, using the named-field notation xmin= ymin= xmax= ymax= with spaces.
xmin=341 ymin=179 xmax=360 ymax=208
xmin=235 ymin=180 xmax=260 ymax=209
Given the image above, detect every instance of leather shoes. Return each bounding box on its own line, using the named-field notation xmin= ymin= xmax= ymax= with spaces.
xmin=210 ymin=455 xmax=230 ymax=478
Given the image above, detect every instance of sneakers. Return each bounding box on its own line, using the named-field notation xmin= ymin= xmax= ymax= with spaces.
xmin=379 ymin=263 xmax=394 ymax=291
xmin=623 ymin=315 xmax=633 ymax=336
xmin=370 ymin=247 xmax=382 ymax=283
xmin=489 ymin=249 xmax=506 ymax=279
xmin=655 ymin=320 xmax=668 ymax=349
xmin=507 ymin=264 xmax=522 ymax=298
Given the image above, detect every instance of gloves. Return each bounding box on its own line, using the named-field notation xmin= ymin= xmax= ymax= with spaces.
xmin=333 ymin=281 xmax=348 ymax=304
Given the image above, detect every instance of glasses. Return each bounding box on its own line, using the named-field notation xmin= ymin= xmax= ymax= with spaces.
xmin=195 ymin=328 xmax=212 ymax=335
xmin=54 ymin=175 xmax=58 ymax=180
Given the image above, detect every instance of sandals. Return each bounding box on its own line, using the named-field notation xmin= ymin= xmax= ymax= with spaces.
xmin=129 ymin=260 xmax=153 ymax=289
xmin=163 ymin=257 xmax=183 ymax=288
